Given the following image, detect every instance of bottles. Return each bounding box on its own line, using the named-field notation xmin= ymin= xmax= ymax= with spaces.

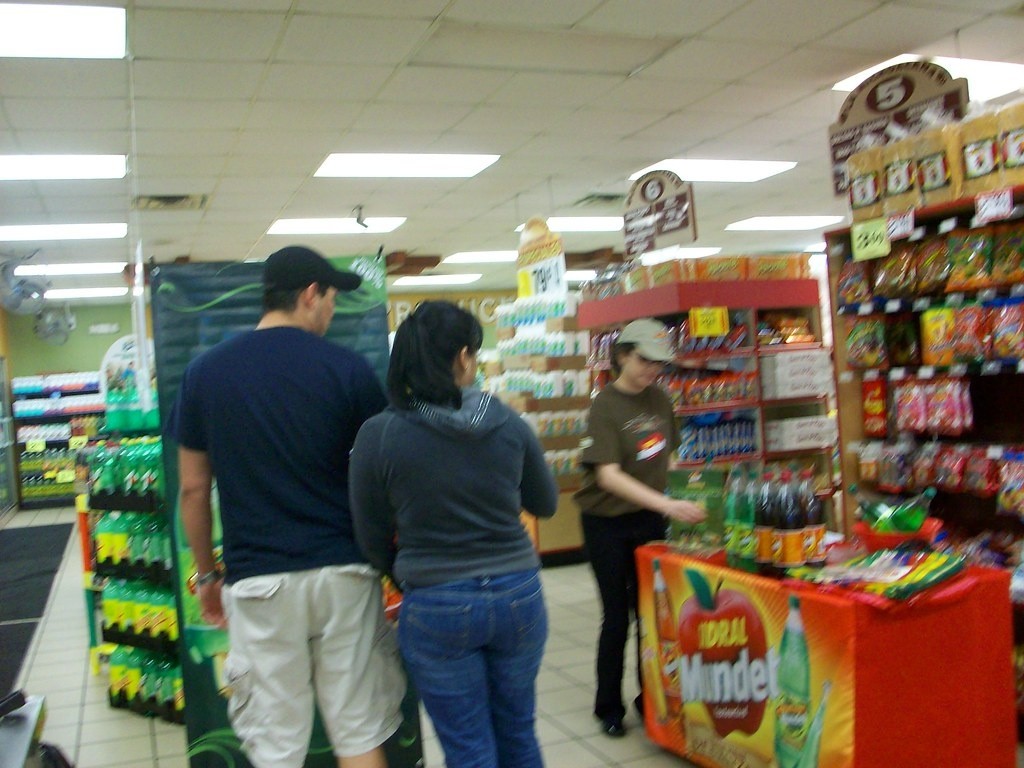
xmin=724 ymin=467 xmax=827 ymax=579
xmin=108 ymin=645 xmax=186 ymax=711
xmin=89 ymin=378 xmax=166 ymax=496
xmin=102 ymin=577 xmax=179 ymax=642
xmin=773 ymin=595 xmax=810 ymax=768
xmin=652 ymin=558 xmax=683 ymax=719
xmin=94 ymin=511 xmax=174 ymax=570
xmin=848 ymin=482 xmax=936 ymax=533
xmin=19 ymin=415 xmax=98 ymax=488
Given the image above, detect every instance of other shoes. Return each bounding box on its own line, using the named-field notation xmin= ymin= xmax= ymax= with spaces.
xmin=605 ymin=721 xmax=627 ymax=738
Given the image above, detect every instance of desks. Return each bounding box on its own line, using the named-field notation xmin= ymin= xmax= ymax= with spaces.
xmin=634 ymin=540 xmax=1017 ymax=768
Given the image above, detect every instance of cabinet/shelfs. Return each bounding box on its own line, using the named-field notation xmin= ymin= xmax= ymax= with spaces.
xmin=824 ymin=182 xmax=1024 ymax=745
xmin=577 ymin=279 xmax=839 ymax=528
xmin=11 ymin=389 xmax=109 ymax=509
xmin=74 ymin=447 xmax=186 ymax=725
xmin=479 ymin=317 xmax=586 ymax=566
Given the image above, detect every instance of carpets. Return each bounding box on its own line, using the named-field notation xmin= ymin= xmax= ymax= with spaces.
xmin=0 ymin=522 xmax=74 ymax=700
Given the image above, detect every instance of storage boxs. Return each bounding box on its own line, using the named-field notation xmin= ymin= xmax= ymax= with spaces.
xmin=576 ymin=253 xmax=810 ymax=300
xmin=662 ymin=466 xmax=732 ymax=555
xmin=757 ymin=349 xmax=834 ymax=398
xmin=766 ymin=412 xmax=838 ymax=449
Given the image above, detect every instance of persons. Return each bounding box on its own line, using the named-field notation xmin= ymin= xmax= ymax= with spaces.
xmin=163 ymin=246 xmax=408 ymax=768
xmin=575 ymin=318 xmax=709 ymax=738
xmin=347 ymin=299 xmax=558 ymax=768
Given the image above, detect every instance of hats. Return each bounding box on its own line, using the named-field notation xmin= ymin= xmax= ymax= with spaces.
xmin=261 ymin=246 xmax=363 ymax=292
xmin=617 ymin=318 xmax=676 ymax=362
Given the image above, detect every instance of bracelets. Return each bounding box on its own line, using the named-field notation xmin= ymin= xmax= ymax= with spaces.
xmin=663 ymin=500 xmax=679 ymax=519
xmin=199 ymin=569 xmax=218 ymax=584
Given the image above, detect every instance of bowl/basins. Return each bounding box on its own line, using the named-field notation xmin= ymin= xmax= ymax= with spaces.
xmin=851 ymin=516 xmax=944 ymax=553
